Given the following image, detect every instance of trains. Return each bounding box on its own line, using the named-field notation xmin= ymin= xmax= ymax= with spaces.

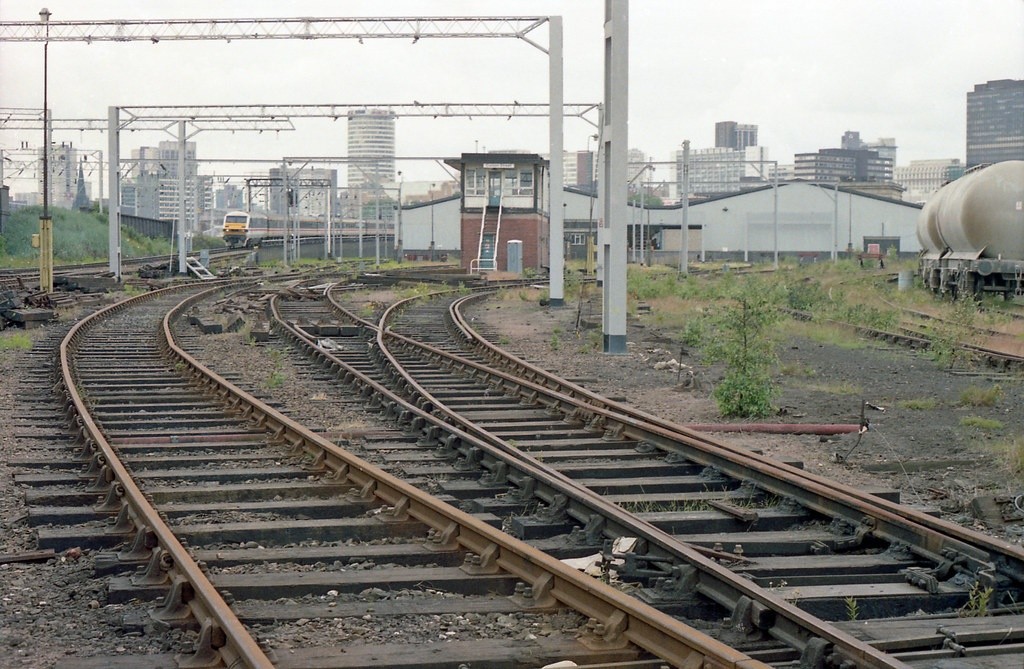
xmin=222 ymin=210 xmax=396 ymax=249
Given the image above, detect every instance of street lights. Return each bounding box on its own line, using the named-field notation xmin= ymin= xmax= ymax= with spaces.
xmin=429 ymin=183 xmax=436 ymax=261
xmin=398 ymin=170 xmax=404 ymax=264
xmin=37 ymin=7 xmax=52 ymax=292
xmin=586 ymin=133 xmax=599 ymax=277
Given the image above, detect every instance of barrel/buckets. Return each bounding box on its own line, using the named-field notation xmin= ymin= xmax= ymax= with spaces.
xmin=899 ymin=269 xmax=914 ymax=291
